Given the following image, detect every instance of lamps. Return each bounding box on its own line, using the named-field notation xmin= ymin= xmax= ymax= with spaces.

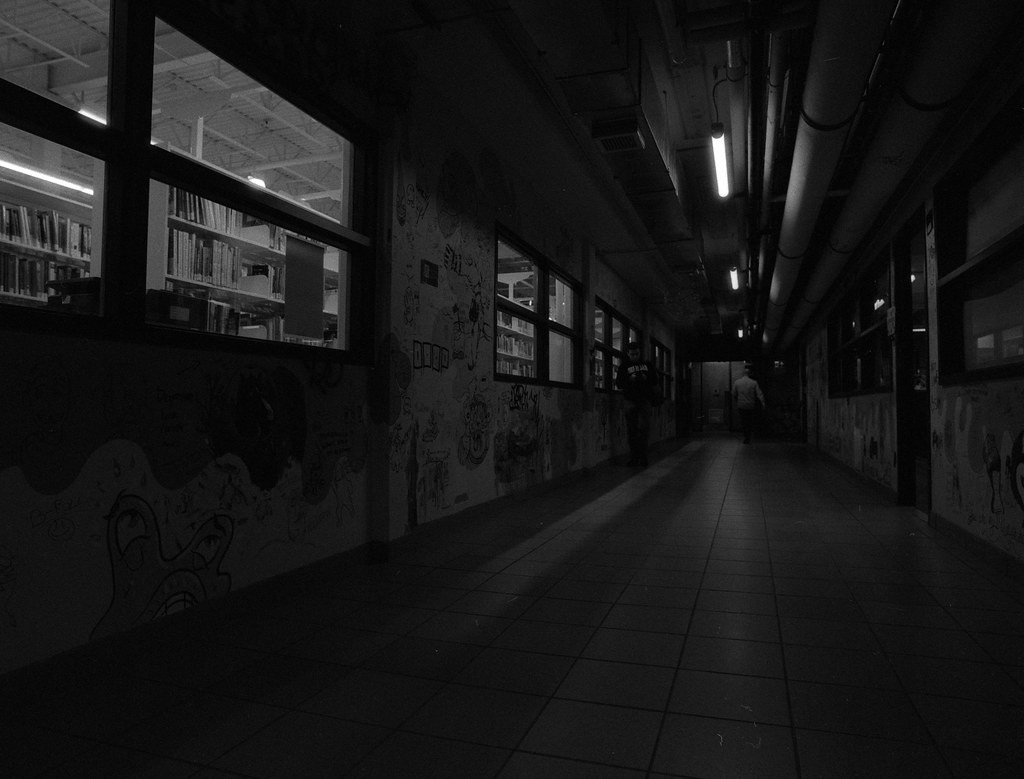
xmin=728 ymin=247 xmax=739 ymax=290
xmin=707 ymin=79 xmax=734 ymax=200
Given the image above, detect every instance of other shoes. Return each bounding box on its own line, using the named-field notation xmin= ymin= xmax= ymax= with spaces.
xmin=744 ymin=438 xmax=750 ymax=444
xmin=626 ymin=459 xmax=648 ymax=471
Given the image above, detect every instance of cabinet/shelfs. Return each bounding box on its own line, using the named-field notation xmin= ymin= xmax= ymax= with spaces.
xmin=496 ymin=294 xmax=535 ymax=378
xmin=0 ymin=141 xmax=340 ymax=348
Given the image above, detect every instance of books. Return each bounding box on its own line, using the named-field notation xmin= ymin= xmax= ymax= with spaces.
xmin=169 ymin=184 xmax=247 ymax=233
xmin=169 ymin=227 xmax=239 ymax=287
xmin=495 ymin=309 xmax=533 ymax=375
xmin=1 ymin=202 xmax=94 ymax=300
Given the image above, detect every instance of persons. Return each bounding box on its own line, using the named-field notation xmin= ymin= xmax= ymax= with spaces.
xmin=616 ymin=343 xmax=657 ymax=466
xmin=732 ymin=367 xmax=765 ymax=443
